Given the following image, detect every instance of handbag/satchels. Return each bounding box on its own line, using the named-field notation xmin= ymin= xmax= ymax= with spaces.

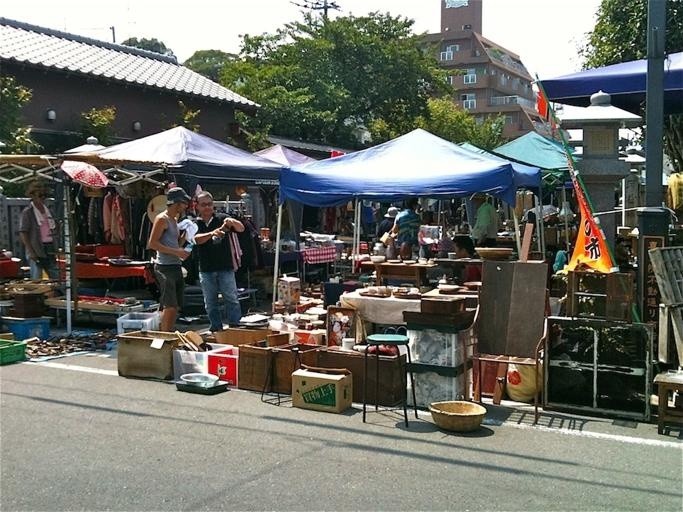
xmin=380 ymin=232 xmax=397 ymax=246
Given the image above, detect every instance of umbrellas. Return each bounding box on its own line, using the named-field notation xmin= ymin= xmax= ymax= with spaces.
xmin=61 ymin=160 xmax=110 ymax=205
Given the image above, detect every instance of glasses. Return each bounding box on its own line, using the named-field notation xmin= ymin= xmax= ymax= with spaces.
xmin=38 ymin=193 xmax=47 ymax=198
xmin=201 ymin=202 xmax=213 ymax=207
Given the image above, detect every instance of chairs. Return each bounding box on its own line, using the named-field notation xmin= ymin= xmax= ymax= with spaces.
xmin=458 ymin=287 xmax=551 ymax=424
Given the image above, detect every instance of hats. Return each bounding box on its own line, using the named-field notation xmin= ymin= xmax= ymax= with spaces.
xmin=470 ymin=192 xmax=486 ymax=200
xmin=384 ymin=207 xmax=400 ymax=218
xmin=167 ymin=188 xmax=191 ymax=205
xmin=26 ymin=184 xmax=46 ymax=195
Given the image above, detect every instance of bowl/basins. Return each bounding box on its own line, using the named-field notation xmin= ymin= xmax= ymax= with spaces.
xmin=474 ymin=247 xmax=513 ymax=261
xmin=428 ymin=400 xmax=487 ymax=433
xmin=180 ymin=372 xmax=220 ymax=388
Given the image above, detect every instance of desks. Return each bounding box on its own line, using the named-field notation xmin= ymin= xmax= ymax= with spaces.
xmin=44 ymin=295 xmax=160 ymax=329
xmin=263 ymin=252 xmax=300 ymax=278
xmin=301 ymin=245 xmax=337 ymax=284
xmin=361 ymin=260 xmax=438 ymax=286
xmin=340 ymin=286 xmax=480 ymax=342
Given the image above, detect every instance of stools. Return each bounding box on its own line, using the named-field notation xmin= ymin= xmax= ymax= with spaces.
xmin=363 ymin=334 xmax=418 ymax=427
xmin=653 ymin=374 xmax=683 ymax=434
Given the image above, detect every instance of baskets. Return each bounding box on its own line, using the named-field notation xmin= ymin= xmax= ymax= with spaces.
xmin=0 ymin=338 xmax=25 ymax=365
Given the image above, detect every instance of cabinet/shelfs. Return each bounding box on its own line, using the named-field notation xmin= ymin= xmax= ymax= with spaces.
xmin=76 ymin=262 xmax=148 ymax=298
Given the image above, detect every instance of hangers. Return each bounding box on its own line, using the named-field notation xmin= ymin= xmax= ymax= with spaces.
xmin=214 ymin=200 xmax=250 ymax=219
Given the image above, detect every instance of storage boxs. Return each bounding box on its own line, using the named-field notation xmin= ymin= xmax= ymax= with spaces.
xmin=238 ymin=340 xmax=290 ymax=392
xmin=3 ymin=320 xmax=50 ymax=342
xmin=272 ymin=344 xmax=321 ymax=394
xmin=207 ymin=347 xmax=239 ymax=389
xmin=291 ymin=364 xmax=354 ymax=414
xmin=316 ymin=348 xmax=407 ymax=407
xmin=117 ymin=330 xmax=184 ymax=381
xmin=116 ymin=312 xmax=160 ymax=335
xmin=172 ymin=343 xmax=232 ymax=382
xmin=14 ymin=294 xmax=45 ymax=319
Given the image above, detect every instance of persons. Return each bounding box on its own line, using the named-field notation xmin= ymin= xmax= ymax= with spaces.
xmin=193 ymin=191 xmax=248 ymax=333
xmin=470 ymin=191 xmax=502 ymax=247
xmin=18 ymin=181 xmax=61 ymax=281
xmin=362 ymin=201 xmax=375 ymax=235
xmin=377 ymin=206 xmax=398 ymax=248
xmin=149 ymin=187 xmax=191 ymax=332
xmin=393 ymin=198 xmax=422 ymax=260
xmin=667 ymin=173 xmax=683 ymax=224
xmin=453 ymin=235 xmax=482 ymax=291
xmin=237 ymin=184 xmax=253 ymax=224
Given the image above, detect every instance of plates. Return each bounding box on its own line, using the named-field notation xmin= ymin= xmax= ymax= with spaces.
xmin=342 ymin=338 xmax=355 ymax=349
xmin=387 ymin=259 xmax=402 ymax=263
xmin=370 ymin=256 xmax=385 ymax=262
xmin=463 ymin=281 xmax=483 ymax=290
xmin=403 ymin=260 xmax=416 ymax=264
xmin=436 ymin=284 xmax=464 ymax=293
